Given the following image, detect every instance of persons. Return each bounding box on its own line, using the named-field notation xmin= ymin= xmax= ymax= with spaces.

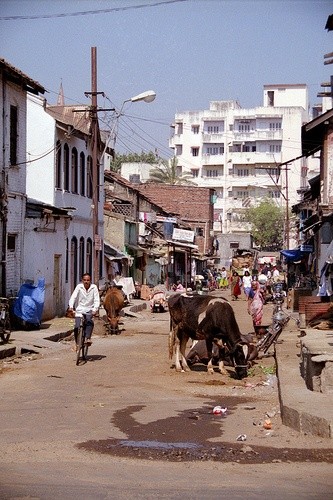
xmin=68 ymin=273 xmax=101 ymax=352
xmin=257 ymin=266 xmax=279 ymax=284
xmin=175 ymin=280 xmax=184 ymax=291
xmin=242 ymin=271 xmax=252 ymax=299
xmin=248 ymin=281 xmax=264 ymax=335
xmin=206 ymin=269 xmax=220 ymax=289
xmin=229 ymin=272 xmax=242 ymax=301
xmin=217 ymin=267 xmax=229 ymax=291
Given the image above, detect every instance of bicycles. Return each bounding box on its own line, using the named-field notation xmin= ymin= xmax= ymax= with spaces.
xmin=0 ymin=296 xmax=20 ymax=343
xmin=256 ymin=302 xmax=301 ymax=354
xmin=68 ymin=310 xmax=99 ymax=366
xmin=272 ymin=282 xmax=286 ymax=315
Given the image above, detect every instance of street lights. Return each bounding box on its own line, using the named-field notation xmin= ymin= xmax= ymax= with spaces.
xmin=90 ymin=90 xmax=157 ymax=287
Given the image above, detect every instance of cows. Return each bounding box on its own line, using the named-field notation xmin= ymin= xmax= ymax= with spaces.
xmin=99 ymin=285 xmax=129 ymax=336
xmin=166 ymin=291 xmax=250 ymax=380
xmin=169 ymin=331 xmax=259 ymax=371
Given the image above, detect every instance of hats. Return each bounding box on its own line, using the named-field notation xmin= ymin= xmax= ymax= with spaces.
xmin=325 ymin=255 xmax=333 ymax=264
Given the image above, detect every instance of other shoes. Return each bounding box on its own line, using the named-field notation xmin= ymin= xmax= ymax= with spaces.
xmin=76 ymin=344 xmax=81 ymax=354
xmin=86 ymin=339 xmax=93 ymax=344
